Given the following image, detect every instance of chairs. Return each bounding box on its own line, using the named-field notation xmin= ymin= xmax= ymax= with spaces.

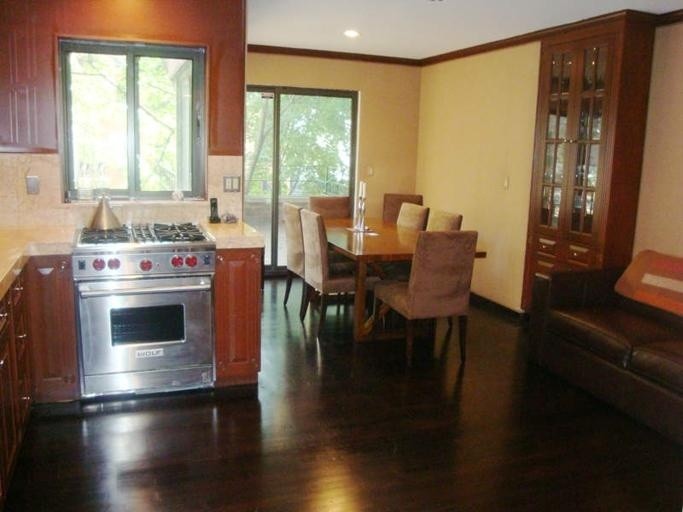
xmin=307 ymin=195 xmax=356 ymax=306
xmin=280 ymin=201 xmax=354 ymax=319
xmin=294 ymin=207 xmax=382 ymax=336
xmin=367 ymin=228 xmax=479 ymax=381
xmin=353 ymin=192 xmax=463 ymax=336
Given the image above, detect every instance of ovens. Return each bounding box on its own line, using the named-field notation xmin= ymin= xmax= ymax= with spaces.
xmin=73 ymin=281 xmax=218 ymax=399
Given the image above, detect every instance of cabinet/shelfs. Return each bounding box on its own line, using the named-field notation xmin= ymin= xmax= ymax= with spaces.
xmin=24 ymin=251 xmax=81 ymax=408
xmin=0 ymin=260 xmax=36 ymax=508
xmin=212 ymin=240 xmax=263 ymax=390
xmin=517 ymin=8 xmax=660 ymax=318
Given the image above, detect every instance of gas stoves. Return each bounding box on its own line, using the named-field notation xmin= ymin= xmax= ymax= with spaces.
xmin=71 ymin=221 xmax=217 ymax=281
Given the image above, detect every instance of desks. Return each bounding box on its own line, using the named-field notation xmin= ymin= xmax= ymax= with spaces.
xmin=319 ymin=215 xmax=487 ymax=344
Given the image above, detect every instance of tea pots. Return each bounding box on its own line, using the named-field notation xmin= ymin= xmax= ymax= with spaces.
xmin=90 ymin=192 xmax=121 ymax=230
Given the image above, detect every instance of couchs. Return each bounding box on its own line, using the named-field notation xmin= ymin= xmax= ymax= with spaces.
xmin=519 ymin=249 xmax=682 ymax=452
